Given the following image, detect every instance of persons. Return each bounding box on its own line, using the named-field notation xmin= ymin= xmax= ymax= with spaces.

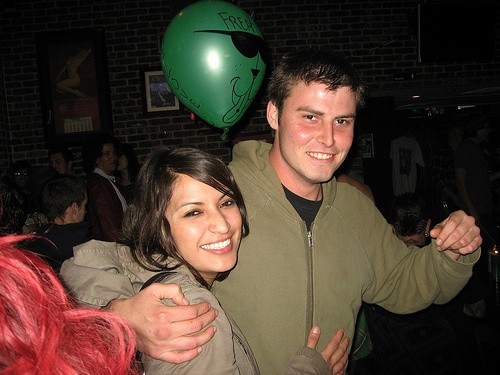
xmin=54 ymin=47 xmax=92 ymax=99
xmin=61 ymin=144 xmax=350 ymax=375
xmin=0 ymin=51 xmax=500 ymax=375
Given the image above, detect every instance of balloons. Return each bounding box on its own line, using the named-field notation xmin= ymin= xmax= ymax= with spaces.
xmin=161 ymin=2 xmax=267 ymax=143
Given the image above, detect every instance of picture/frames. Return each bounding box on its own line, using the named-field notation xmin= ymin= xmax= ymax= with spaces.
xmin=144 ymin=71 xmax=180 ymax=112
xmin=35 ymin=30 xmax=115 ymax=150
xmin=338 ymin=95 xmax=395 ymax=172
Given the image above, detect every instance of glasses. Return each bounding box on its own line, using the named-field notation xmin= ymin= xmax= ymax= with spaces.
xmin=13 ymin=171 xmax=29 ymax=176
